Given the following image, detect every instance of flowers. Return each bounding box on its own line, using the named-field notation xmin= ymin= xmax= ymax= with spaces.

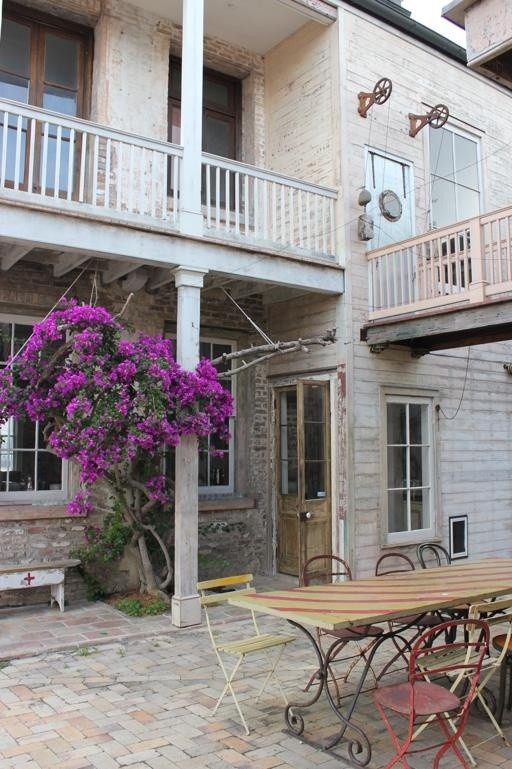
xmin=0 ymin=299 xmax=236 ymax=566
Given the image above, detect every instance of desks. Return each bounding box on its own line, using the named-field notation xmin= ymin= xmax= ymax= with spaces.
xmin=228 ymin=557 xmax=512 ymax=766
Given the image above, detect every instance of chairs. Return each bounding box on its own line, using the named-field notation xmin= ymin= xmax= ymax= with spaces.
xmin=377 ymin=618 xmax=490 ymax=768
xmin=302 ymin=555 xmax=387 ymax=707
xmin=373 ymin=552 xmax=443 ymax=676
xmin=410 ymin=599 xmax=512 ymax=768
xmin=197 ymin=574 xmax=298 ymax=734
xmin=417 ymin=545 xmax=488 ymax=646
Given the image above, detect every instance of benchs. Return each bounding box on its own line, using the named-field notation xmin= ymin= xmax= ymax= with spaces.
xmin=0 ymin=558 xmax=81 ymax=612
